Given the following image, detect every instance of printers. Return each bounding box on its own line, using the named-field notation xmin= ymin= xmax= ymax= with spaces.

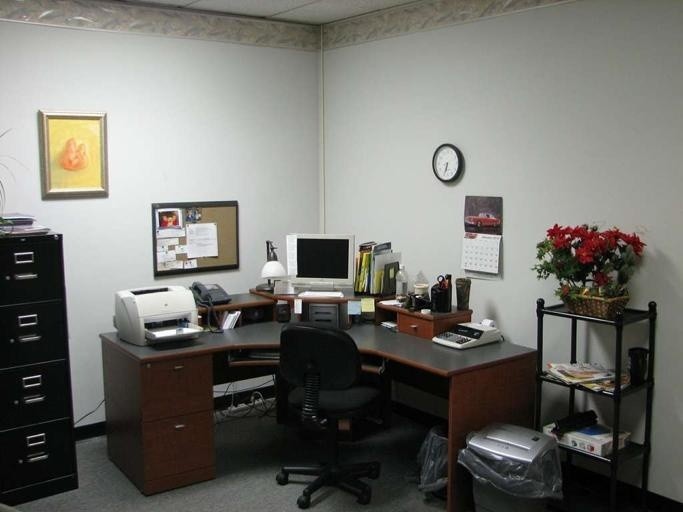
xmin=113 ymin=286 xmax=204 ymax=347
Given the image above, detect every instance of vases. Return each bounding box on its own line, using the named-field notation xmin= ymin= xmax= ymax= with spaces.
xmin=560 ymin=292 xmax=631 ymax=320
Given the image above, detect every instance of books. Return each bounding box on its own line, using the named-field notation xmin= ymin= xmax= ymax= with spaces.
xmin=354 ymin=240 xmax=403 ymax=296
xmin=543 ymin=359 xmax=630 ymax=396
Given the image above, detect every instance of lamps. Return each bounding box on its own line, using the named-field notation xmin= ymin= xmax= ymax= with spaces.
xmin=257 ymin=240 xmax=285 ymax=291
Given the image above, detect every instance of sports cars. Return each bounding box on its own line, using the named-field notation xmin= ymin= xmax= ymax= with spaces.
xmin=465 ymin=212 xmax=500 ymax=228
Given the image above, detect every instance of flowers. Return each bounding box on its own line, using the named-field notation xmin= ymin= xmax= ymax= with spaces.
xmin=531 ymin=222 xmax=647 ymax=298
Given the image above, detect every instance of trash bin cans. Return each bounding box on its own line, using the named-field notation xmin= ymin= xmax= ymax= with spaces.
xmin=469 ymin=423 xmax=557 ymax=512
xmin=428 ymin=425 xmax=449 ymax=500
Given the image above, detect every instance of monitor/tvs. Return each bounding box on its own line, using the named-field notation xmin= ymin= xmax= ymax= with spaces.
xmin=286 ymin=233 xmax=355 ymax=298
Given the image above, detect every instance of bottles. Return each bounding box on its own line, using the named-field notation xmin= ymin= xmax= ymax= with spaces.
xmin=394 ymin=264 xmax=408 ymax=295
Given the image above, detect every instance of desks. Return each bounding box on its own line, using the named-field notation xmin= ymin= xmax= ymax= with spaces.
xmin=102 ymin=288 xmax=536 ymax=512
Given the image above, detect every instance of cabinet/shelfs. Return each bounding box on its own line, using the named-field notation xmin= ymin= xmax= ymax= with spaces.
xmin=0 ymin=233 xmax=80 ymax=505
xmin=533 ymin=300 xmax=658 ymax=512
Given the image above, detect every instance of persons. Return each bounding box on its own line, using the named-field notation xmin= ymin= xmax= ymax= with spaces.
xmin=161 ymin=214 xmax=179 ymax=227
xmin=186 ymin=210 xmax=194 ymax=221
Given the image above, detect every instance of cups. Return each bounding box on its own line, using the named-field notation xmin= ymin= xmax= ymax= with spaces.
xmin=455 ymin=278 xmax=472 ymax=311
xmin=414 ymin=284 xmax=429 ymax=299
xmin=627 ymin=347 xmax=649 ymax=383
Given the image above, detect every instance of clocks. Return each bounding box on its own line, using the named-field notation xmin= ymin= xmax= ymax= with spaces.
xmin=432 ymin=144 xmax=463 ymax=183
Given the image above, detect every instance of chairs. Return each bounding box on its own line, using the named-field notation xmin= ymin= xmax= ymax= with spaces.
xmin=275 ymin=325 xmax=391 ymax=509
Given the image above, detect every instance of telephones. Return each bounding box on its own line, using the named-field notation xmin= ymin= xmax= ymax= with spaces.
xmin=189 ymin=281 xmax=232 ymax=333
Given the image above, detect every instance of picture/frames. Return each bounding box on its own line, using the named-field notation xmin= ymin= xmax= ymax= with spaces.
xmin=36 ymin=109 xmax=109 ymax=199
xmin=152 ymin=201 xmax=239 ymax=276
